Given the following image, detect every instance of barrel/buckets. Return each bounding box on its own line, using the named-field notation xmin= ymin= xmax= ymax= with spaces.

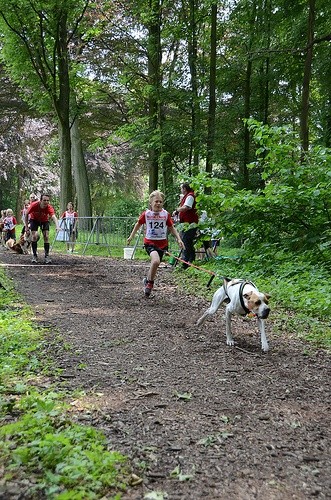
xmin=124 ymin=248 xmax=134 ymax=259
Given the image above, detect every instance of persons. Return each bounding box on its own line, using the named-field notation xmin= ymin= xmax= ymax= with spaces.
xmin=0 ymin=192 xmax=39 ymax=255
xmin=25 ymin=194 xmax=61 ymax=263
xmin=172 ymin=181 xmax=199 ymax=268
xmin=60 ymin=201 xmax=78 ymax=253
xmin=127 ymin=190 xmax=186 ymax=297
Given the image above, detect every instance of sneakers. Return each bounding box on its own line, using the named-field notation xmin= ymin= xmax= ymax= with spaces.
xmin=142 ymin=277 xmax=155 ymax=296
xmin=32 ymin=256 xmax=38 ymax=263
xmin=44 ymin=256 xmax=52 ymax=264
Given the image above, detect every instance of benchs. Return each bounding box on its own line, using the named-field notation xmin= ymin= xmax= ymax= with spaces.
xmin=49 ymin=217 xmax=184 ymax=260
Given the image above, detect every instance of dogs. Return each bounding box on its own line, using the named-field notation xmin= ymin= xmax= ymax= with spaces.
xmin=196 ymin=279 xmax=272 ymax=352
